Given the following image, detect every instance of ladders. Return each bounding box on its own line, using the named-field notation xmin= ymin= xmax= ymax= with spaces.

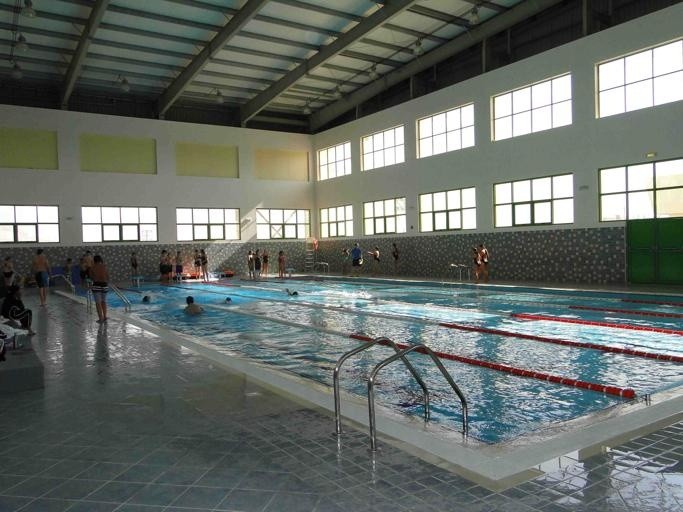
xmin=304 ymin=237 xmax=314 ymax=274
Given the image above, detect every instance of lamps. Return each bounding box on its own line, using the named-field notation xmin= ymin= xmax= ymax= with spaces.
xmin=10 ymin=0 xmax=35 ymax=79
xmin=303 ymin=4 xmax=481 ymax=116
xmin=117 ymin=74 xmax=129 ymax=93
xmin=215 ymin=90 xmax=224 ymax=104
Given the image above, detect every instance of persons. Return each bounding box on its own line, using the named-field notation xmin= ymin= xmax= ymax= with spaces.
xmin=88 ymin=255 xmax=109 ymax=322
xmin=130 ymin=252 xmax=139 ymax=285
xmin=75 ymin=258 xmax=91 ymax=289
xmin=479 ymin=243 xmax=490 ymax=284
xmin=182 ymin=295 xmax=204 ymax=314
xmin=391 ymin=243 xmax=401 ymax=277
xmin=174 ymin=251 xmax=183 ymax=282
xmin=252 ymin=249 xmax=261 ymax=277
xmin=78 ymin=249 xmax=94 ymax=279
xmin=30 ymin=247 xmax=50 ymax=307
xmin=74 ymin=254 xmax=91 ymax=279
xmin=224 ymin=297 xmax=232 ymax=304
xmin=245 ymin=250 xmax=256 ymax=279
xmin=159 ymin=249 xmax=169 ymax=283
xmin=277 ymin=250 xmax=283 ymax=278
xmin=165 ymin=252 xmax=175 ymax=280
xmin=61 ymin=257 xmax=79 ymax=289
xmin=0 ymin=255 xmax=21 ymax=286
xmin=193 ymin=248 xmax=201 ymax=281
xmin=284 ymin=287 xmax=298 ymax=296
xmin=1 ymin=284 xmax=37 ymax=336
xmin=349 ymin=242 xmax=362 ymax=278
xmin=141 ymin=295 xmax=150 ymax=303
xmin=261 ymin=248 xmax=268 ymax=277
xmin=469 ymin=248 xmax=481 ymax=283
xmin=198 ymin=248 xmax=209 ymax=281
xmin=279 ymin=250 xmax=287 ymax=278
xmin=368 ymin=245 xmax=380 ymax=278
xmin=336 ymin=246 xmax=349 ymax=276
